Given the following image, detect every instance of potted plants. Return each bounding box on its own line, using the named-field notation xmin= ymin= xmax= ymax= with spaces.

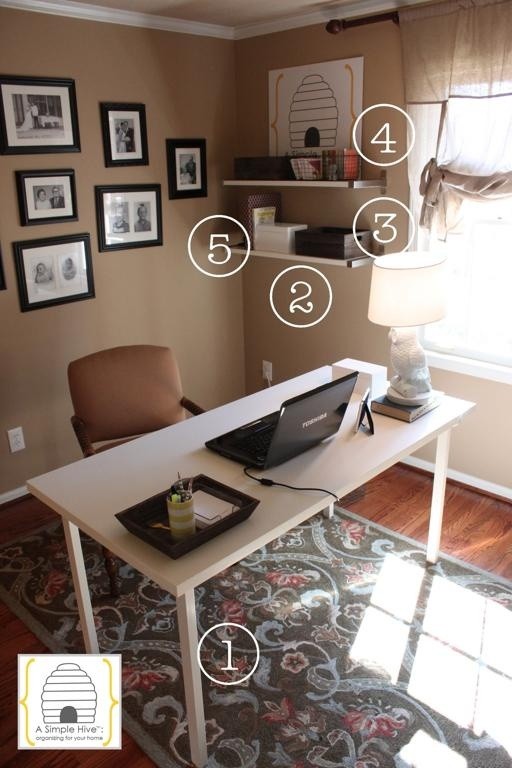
xmin=100 ymin=102 xmax=152 ymax=168
xmin=1 ymin=73 xmax=85 ymax=156
xmin=94 ymin=183 xmax=166 ymax=253
xmin=16 ymin=168 xmax=81 ymax=227
xmin=166 ymin=136 xmax=210 ymax=200
xmin=12 ymin=233 xmax=102 ymax=313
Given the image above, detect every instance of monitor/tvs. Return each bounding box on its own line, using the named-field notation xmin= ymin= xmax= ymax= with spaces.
xmin=371 ymin=388 xmax=445 ymax=424
xmin=252 ymin=207 xmax=275 ymax=249
xmin=291 ymin=158 xmax=321 ymax=179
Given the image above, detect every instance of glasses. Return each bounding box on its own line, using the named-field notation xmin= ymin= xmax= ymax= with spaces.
xmin=365 ymin=252 xmax=451 ymax=406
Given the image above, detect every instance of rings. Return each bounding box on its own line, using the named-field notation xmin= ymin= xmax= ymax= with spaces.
xmin=164 ymin=470 xmax=194 ymax=506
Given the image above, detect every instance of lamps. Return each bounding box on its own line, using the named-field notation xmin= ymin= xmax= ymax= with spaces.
xmin=52 ymin=344 xmax=208 ymax=599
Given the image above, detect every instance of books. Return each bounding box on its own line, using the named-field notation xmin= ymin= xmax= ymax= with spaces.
xmin=253 ymin=223 xmax=307 ymax=257
xmin=233 ymin=152 xmax=361 ymax=179
xmin=297 ymin=227 xmax=372 ymax=260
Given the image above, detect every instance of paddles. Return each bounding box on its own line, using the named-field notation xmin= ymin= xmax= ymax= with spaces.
xmin=0 ymin=491 xmax=511 ymax=768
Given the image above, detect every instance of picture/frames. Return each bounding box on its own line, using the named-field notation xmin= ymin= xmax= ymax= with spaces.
xmin=26 ymin=365 xmax=478 ymax=768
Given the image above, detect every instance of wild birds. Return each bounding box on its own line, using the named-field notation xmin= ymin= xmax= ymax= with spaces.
xmin=204 ymin=371 xmax=359 ymax=473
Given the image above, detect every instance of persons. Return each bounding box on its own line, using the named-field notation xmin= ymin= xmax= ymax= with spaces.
xmin=32 ymin=102 xmax=40 ymax=129
xmin=50 ymin=187 xmax=64 ymax=208
xmin=118 ymin=120 xmax=134 ymax=152
xmin=35 ymin=264 xmax=52 ymax=283
xmin=18 ymin=102 xmax=33 ymax=131
xmin=36 ymin=189 xmax=50 ymax=209
xmin=112 ymin=206 xmax=129 ymax=233
xmin=187 ymin=155 xmax=196 ymax=184
xmin=135 ymin=205 xmax=151 ymax=231
xmin=63 ymin=259 xmax=76 ymax=280
xmin=180 ymin=167 xmax=192 ymax=185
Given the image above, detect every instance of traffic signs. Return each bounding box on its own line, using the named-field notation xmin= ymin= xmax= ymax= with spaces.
xmin=9 ymin=427 xmax=26 ymax=453
xmin=262 ymin=360 xmax=273 ymax=381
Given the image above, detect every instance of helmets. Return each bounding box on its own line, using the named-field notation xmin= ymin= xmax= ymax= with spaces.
xmin=114 ymin=473 xmax=261 ymax=561
